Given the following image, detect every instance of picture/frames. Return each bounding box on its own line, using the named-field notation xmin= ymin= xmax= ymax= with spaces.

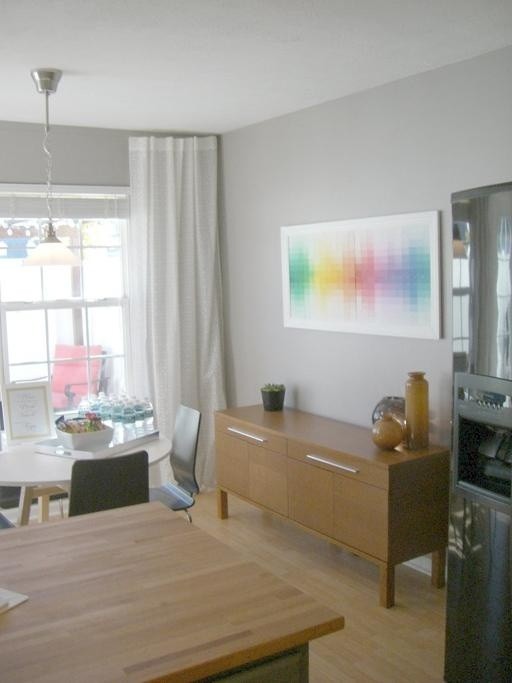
xmin=279 ymin=209 xmax=442 ymax=338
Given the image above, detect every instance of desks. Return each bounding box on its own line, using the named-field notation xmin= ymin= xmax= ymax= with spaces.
xmin=0 ymin=501 xmax=345 ymax=682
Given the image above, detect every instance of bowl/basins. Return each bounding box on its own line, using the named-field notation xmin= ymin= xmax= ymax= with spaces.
xmin=55 ymin=421 xmax=113 ymax=449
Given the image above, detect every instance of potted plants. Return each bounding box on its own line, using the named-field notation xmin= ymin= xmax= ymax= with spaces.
xmin=259 ymin=382 xmax=286 ymax=410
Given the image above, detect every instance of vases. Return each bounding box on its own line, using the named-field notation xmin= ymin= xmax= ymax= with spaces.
xmin=369 ymin=371 xmax=433 ymax=452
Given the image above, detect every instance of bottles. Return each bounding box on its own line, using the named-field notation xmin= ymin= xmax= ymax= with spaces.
xmin=404 ymin=369 xmax=431 ymax=451
xmin=81 ymin=391 xmax=155 ymax=431
xmin=371 ymin=395 xmax=407 ymax=438
xmin=370 ymin=410 xmax=404 ymax=451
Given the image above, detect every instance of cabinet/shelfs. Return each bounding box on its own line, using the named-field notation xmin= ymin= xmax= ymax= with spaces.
xmin=215 ymin=414 xmax=450 ymax=611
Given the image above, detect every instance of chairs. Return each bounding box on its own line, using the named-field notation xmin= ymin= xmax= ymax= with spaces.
xmin=47 ymin=342 xmax=109 ymax=411
xmin=0 ymin=402 xmax=203 ymax=529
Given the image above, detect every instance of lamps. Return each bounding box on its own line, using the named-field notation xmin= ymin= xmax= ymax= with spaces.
xmin=20 ymin=68 xmax=77 ymax=268
